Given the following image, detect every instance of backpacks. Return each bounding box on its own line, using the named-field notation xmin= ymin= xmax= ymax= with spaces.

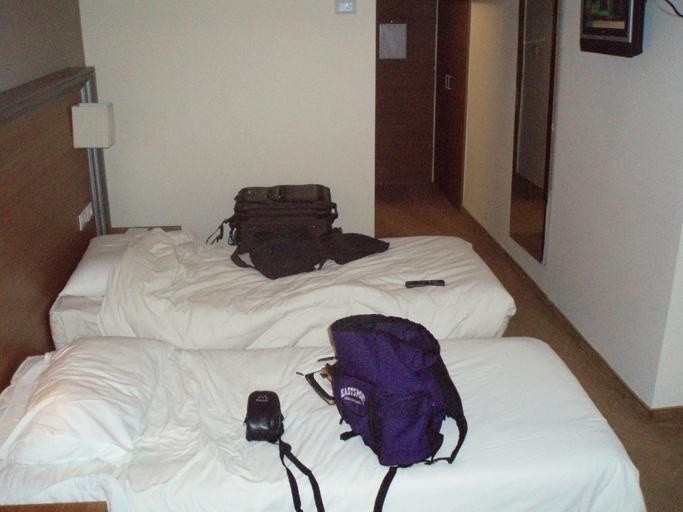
xmin=229 ymin=184 xmax=338 ymax=279
xmin=328 ymin=314 xmax=468 ymax=467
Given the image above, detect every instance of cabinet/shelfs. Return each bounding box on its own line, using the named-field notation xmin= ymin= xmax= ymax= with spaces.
xmin=436 ymin=1 xmax=472 ymax=211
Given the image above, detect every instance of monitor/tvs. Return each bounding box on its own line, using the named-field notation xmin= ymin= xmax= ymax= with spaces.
xmin=579 ymin=0 xmax=645 ymax=57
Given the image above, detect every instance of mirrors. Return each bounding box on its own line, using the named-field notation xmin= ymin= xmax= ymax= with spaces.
xmin=509 ymin=1 xmax=559 ymax=262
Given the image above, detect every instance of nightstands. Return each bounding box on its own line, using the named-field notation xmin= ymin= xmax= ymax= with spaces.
xmin=0 ymin=501 xmax=108 ymax=512
xmin=108 ymin=225 xmax=182 ymax=234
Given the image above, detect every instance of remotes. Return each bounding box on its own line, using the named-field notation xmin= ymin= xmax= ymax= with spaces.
xmin=405 ymin=280 xmax=445 ymax=288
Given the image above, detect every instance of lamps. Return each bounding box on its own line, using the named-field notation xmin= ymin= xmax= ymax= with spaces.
xmin=71 ymin=103 xmax=116 ymax=236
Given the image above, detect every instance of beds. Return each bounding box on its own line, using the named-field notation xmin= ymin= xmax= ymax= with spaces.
xmin=1 ymin=336 xmax=646 ymax=512
xmin=47 ymin=234 xmax=518 ymax=348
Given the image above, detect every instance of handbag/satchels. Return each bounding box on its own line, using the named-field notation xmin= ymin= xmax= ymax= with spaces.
xmin=246 ymin=391 xmax=284 ymax=442
